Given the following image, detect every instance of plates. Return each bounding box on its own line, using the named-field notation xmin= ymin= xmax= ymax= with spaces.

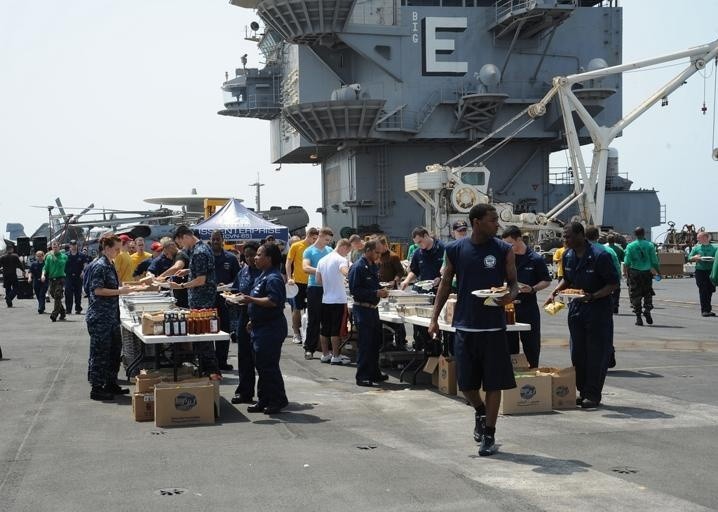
xmin=157 ymin=282 xmax=170 ymax=288
xmin=220 ymin=292 xmax=236 ymax=299
xmin=380 ymin=283 xmax=391 ymax=287
xmin=284 ymin=283 xmax=299 ymax=299
xmin=558 ymin=293 xmax=585 ymax=299
xmin=470 ymin=288 xmax=509 ymax=298
xmin=413 ymin=280 xmax=434 ymax=287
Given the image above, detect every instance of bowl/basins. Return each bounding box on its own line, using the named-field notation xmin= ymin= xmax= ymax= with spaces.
xmin=700 ymin=257 xmax=712 ymax=261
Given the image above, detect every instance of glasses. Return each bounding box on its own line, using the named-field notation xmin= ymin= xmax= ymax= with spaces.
xmin=156 ymin=249 xmax=163 ymax=252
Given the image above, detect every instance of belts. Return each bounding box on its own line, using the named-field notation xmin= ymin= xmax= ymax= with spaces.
xmin=353 ymin=302 xmax=377 ymax=310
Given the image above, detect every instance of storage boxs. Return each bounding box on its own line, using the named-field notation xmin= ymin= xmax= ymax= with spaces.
xmin=130 ymin=368 xmax=222 ymax=429
xmin=439 ymin=353 xmax=576 ymax=417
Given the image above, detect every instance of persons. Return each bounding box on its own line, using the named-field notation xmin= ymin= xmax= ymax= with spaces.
xmin=283 ymin=220 xmax=468 ymax=386
xmin=85 ymin=220 xmax=260 ymax=407
xmin=2 ymin=245 xmax=26 ymax=310
xmin=502 ymin=224 xmax=552 ymax=369
xmin=541 ymin=222 xmax=620 ymax=407
xmin=554 ymin=220 xmax=663 ymax=368
xmin=427 ymin=203 xmax=519 ymax=458
xmin=27 ymin=240 xmax=86 ymax=322
xmin=685 ymin=229 xmax=718 ymax=318
xmin=239 ymin=241 xmax=289 ymax=414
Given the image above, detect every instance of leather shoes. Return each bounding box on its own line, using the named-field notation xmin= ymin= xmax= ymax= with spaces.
xmin=104 ymin=384 xmax=129 ymax=395
xmin=247 ymin=400 xmax=265 ymax=412
xmin=356 ymin=378 xmax=373 ymax=387
xmin=90 ymin=387 xmax=113 ymax=400
xmin=264 ymin=398 xmax=288 ymax=414
xmin=370 ymin=371 xmax=388 ymax=383
xmin=231 ymin=394 xmax=252 ymax=403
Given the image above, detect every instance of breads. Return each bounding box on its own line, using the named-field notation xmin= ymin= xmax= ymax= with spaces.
xmin=491 ymin=285 xmax=507 ymax=294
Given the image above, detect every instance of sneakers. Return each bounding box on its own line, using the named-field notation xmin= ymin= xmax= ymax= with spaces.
xmin=229 ymin=332 xmax=237 ymax=342
xmin=479 ymin=435 xmax=500 ymax=456
xmin=474 ymin=413 xmax=486 ymax=442
xmin=708 ymin=312 xmax=716 ymax=316
xmin=59 ymin=315 xmax=66 ymax=319
xmin=50 ymin=316 xmax=56 ymax=322
xmin=304 ymin=352 xmax=312 ymax=360
xmin=331 ymin=355 xmax=350 ymax=366
xmin=576 ymin=397 xmax=586 ymax=405
xmin=7 ymin=304 xmax=12 ymax=307
xmin=38 ymin=309 xmax=44 ymax=314
xmin=581 ymin=399 xmax=599 ymax=408
xmin=218 ymin=365 xmax=232 ymax=371
xmin=75 ymin=311 xmax=81 ymax=314
xmin=702 ymin=312 xmax=710 ymax=317
xmin=635 ymin=314 xmax=643 ymax=326
xmin=320 ymin=351 xmax=333 ymax=363
xmin=46 ymin=297 xmax=49 ymax=302
xmin=65 ymin=310 xmax=71 ymax=314
xmin=292 ymin=333 xmax=302 ymax=344
xmin=643 ymin=309 xmax=653 ymax=324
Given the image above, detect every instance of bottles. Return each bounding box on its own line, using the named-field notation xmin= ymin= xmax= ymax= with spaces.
xmin=504 ymin=303 xmax=515 ymax=325
xmin=162 ymin=308 xmax=220 ymax=336
xmin=655 ymin=275 xmax=660 ymax=282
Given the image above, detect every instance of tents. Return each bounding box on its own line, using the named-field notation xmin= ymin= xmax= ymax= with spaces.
xmin=189 ymin=197 xmax=289 ymax=253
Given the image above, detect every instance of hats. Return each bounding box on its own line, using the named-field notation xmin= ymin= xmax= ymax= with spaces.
xmin=118 ymin=235 xmax=133 ymax=241
xmin=453 ymin=220 xmax=468 ymax=231
xmin=70 ymin=240 xmax=77 ymax=244
xmin=151 ymin=242 xmax=164 ymax=251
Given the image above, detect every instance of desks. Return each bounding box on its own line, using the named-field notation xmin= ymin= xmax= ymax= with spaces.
xmin=339 ymin=290 xmax=531 ymax=387
xmin=120 ymin=279 xmax=229 ymax=380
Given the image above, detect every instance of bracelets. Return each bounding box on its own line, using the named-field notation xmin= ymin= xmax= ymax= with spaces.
xmin=530 ymin=286 xmax=534 ymax=292
